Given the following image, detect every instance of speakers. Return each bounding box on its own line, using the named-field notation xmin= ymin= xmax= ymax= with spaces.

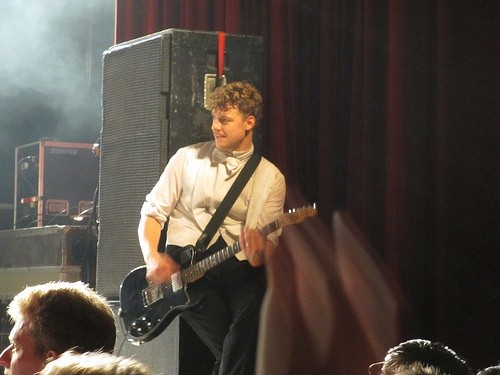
xmin=103 ymin=300 xmax=216 ymax=375
xmin=95 ymin=28 xmax=264 ymax=301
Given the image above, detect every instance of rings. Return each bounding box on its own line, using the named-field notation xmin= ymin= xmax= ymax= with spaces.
xmin=245 ymin=242 xmax=249 ymax=247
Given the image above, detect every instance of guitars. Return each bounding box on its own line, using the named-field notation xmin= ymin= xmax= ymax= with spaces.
xmin=117 ymin=203 xmax=316 ymax=347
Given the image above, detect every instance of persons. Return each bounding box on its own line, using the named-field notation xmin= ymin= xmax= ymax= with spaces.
xmin=137 ymin=81 xmax=286 ymax=375
xmin=380 ymin=339 xmax=472 ymax=375
xmin=0 ymin=280 xmax=149 ymax=375
